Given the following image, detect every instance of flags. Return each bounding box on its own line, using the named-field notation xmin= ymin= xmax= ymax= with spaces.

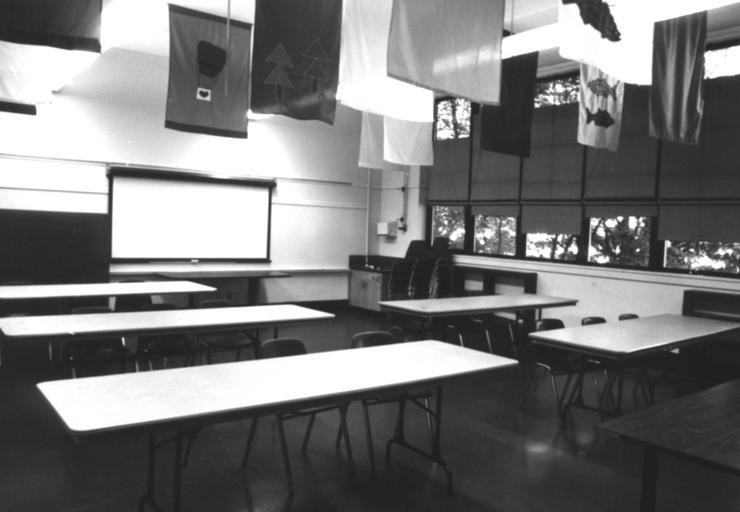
xmin=0 ymin=0 xmax=103 ymax=55
xmin=335 ymin=0 xmax=435 ymax=125
xmin=164 ymin=2 xmax=251 ymax=139
xmin=480 ymin=29 xmax=539 ymax=158
xmin=248 ymin=0 xmax=343 ymax=125
xmin=648 ymin=10 xmax=708 ymax=145
xmin=386 ymin=0 xmax=507 ymax=107
xmin=357 ymin=111 xmax=408 ymax=173
xmin=382 ymin=115 xmax=435 ymax=167
xmin=576 ymin=60 xmax=626 ymax=155
xmin=557 ymin=0 xmax=659 ymax=86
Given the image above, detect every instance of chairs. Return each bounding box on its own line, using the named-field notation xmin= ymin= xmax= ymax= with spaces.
xmin=385 ymin=236 xmax=457 ymax=313
xmin=336 ymin=332 xmax=435 ymax=476
xmin=621 ymin=315 xmax=685 ymax=406
xmin=572 ymin=316 xmax=647 ymax=417
xmin=240 ymin=340 xmax=356 ymax=496
xmin=518 ymin=320 xmax=604 ymax=434
xmin=0 ymin=270 xmax=579 ymax=368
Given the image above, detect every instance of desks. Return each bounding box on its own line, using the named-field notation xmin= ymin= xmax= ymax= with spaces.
xmin=37 ymin=339 xmax=520 ymax=507
xmin=594 ymin=379 xmax=738 ymax=510
xmin=531 ymin=312 xmax=727 ymax=448
